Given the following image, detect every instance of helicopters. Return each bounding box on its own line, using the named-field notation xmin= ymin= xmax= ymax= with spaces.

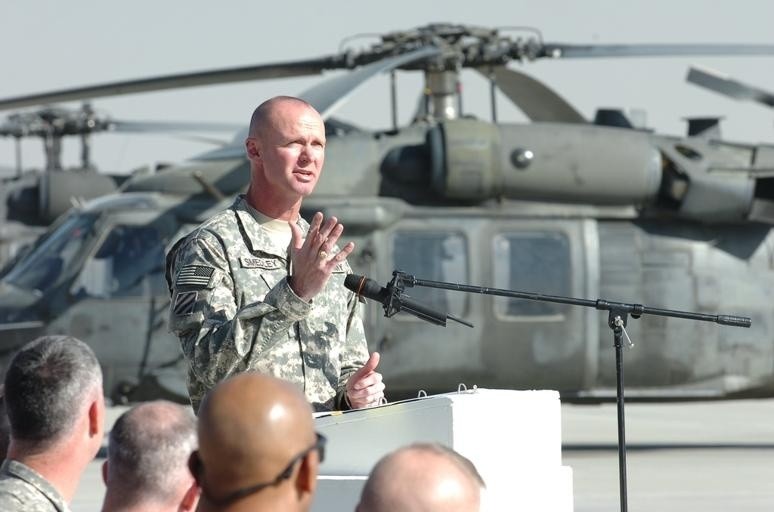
xmin=0 ymin=102 xmax=250 ymax=279
xmin=0 ymin=21 xmax=773 ymax=468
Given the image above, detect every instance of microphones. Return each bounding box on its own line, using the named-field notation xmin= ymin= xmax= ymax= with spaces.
xmin=343 ymin=274 xmax=446 ymax=320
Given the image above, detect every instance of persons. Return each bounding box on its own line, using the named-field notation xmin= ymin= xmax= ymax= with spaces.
xmin=353 ymin=439 xmax=487 ymax=512
xmin=160 ymin=97 xmax=386 ymax=422
xmin=101 ymin=399 xmax=204 ymax=512
xmin=187 ymin=370 xmax=322 ymax=511
xmin=0 ymin=333 xmax=107 ymax=510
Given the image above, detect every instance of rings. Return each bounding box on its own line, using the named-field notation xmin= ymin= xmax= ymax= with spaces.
xmin=317 ymin=250 xmax=327 ymax=260
